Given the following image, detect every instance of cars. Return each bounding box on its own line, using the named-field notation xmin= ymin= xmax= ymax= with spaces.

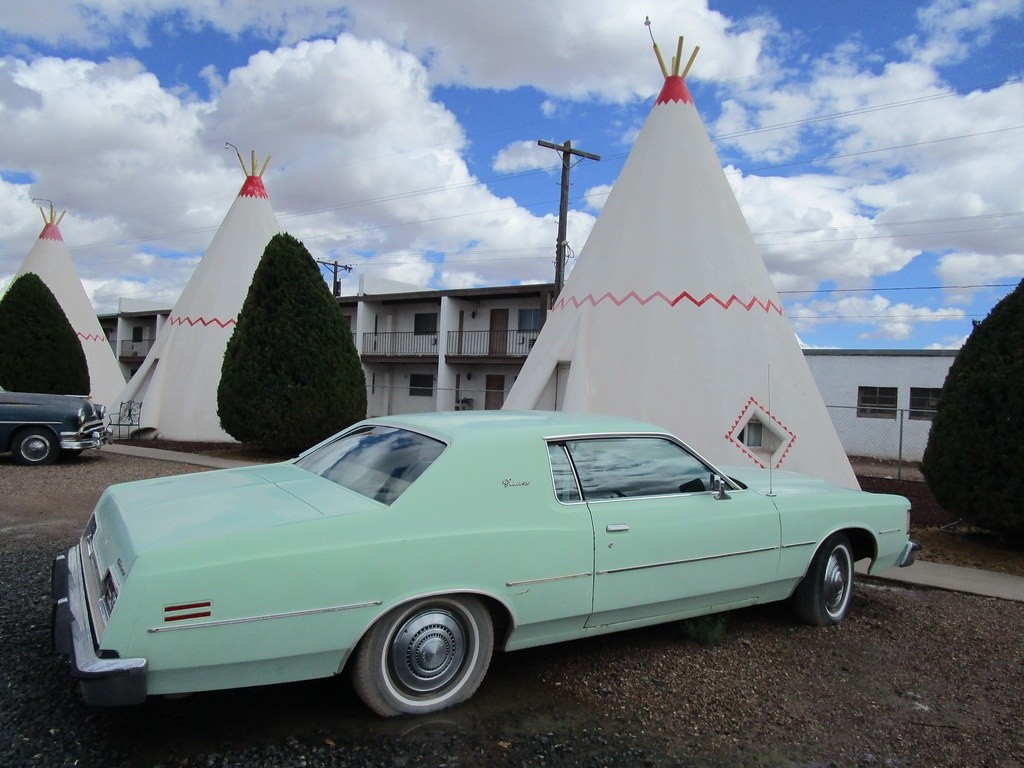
xmin=0 ymin=386 xmax=115 ymax=466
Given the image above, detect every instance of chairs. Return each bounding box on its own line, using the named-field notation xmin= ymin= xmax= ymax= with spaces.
xmin=106 ymin=400 xmax=142 ymax=442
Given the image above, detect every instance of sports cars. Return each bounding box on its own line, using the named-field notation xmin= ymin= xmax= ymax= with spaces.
xmin=53 ymin=410 xmax=923 ymax=723
